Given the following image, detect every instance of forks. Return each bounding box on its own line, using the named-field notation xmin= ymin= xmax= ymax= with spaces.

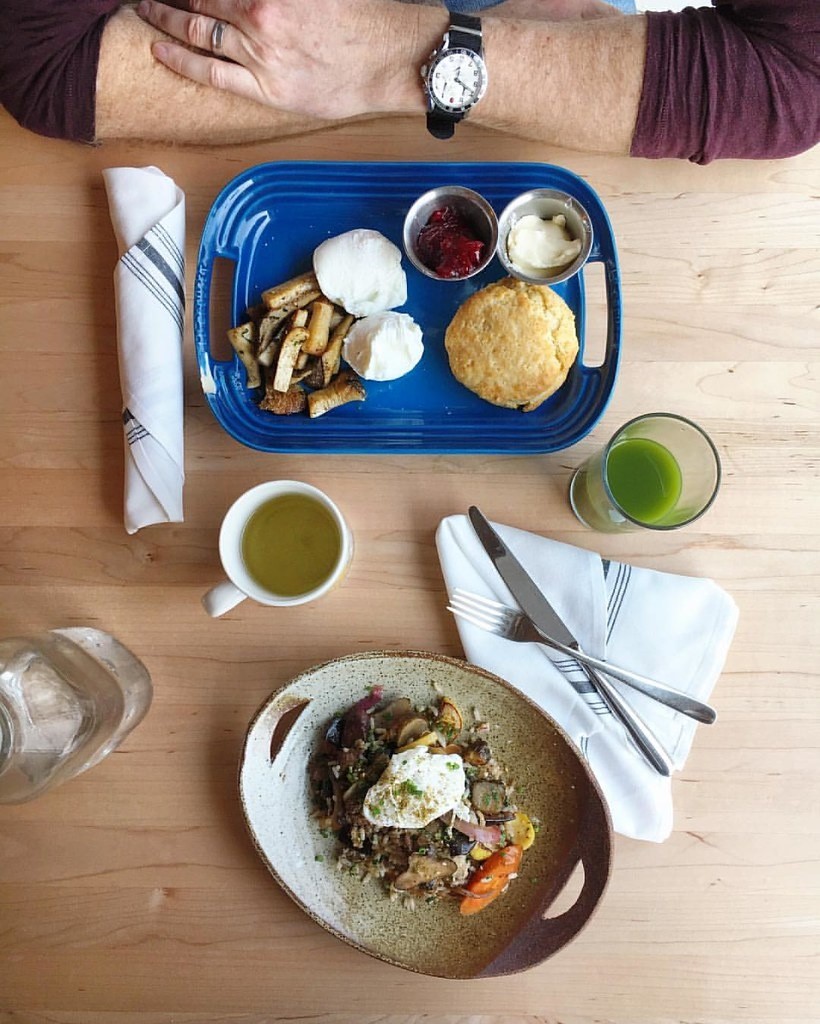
xmin=446 ymin=588 xmax=716 ymax=724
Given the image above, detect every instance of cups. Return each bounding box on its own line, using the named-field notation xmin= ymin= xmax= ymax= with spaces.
xmin=567 ymin=413 xmax=722 ymax=534
xmin=201 ymin=480 xmax=351 ymax=618
xmin=498 ymin=188 xmax=594 ymax=285
xmin=402 ymin=186 xmax=498 ymax=280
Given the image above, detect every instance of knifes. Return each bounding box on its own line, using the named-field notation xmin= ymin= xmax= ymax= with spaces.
xmin=468 ymin=504 xmax=672 ymax=777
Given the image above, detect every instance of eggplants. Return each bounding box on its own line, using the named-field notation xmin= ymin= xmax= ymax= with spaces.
xmin=310 ymin=684 xmax=538 ymax=916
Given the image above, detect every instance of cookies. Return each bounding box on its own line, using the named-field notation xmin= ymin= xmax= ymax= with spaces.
xmin=443 ymin=277 xmax=581 ymax=413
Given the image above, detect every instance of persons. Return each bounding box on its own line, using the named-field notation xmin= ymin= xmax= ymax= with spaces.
xmin=0 ymin=0 xmax=820 ymax=166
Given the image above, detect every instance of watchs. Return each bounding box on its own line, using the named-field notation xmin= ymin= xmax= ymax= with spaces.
xmin=420 ymin=8 xmax=487 ymax=140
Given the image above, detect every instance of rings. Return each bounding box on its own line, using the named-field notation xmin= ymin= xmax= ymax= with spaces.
xmin=211 ymin=19 xmax=230 ymax=58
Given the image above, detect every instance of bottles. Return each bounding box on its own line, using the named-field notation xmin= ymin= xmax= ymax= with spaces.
xmin=0 ymin=626 xmax=153 ymax=806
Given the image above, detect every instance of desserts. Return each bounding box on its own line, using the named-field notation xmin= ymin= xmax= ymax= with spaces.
xmin=506 ymin=212 xmax=582 ymax=280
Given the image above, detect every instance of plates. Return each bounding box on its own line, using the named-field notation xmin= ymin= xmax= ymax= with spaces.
xmin=237 ymin=650 xmax=615 ymax=980
xmin=192 ymin=161 xmax=621 ymax=455
